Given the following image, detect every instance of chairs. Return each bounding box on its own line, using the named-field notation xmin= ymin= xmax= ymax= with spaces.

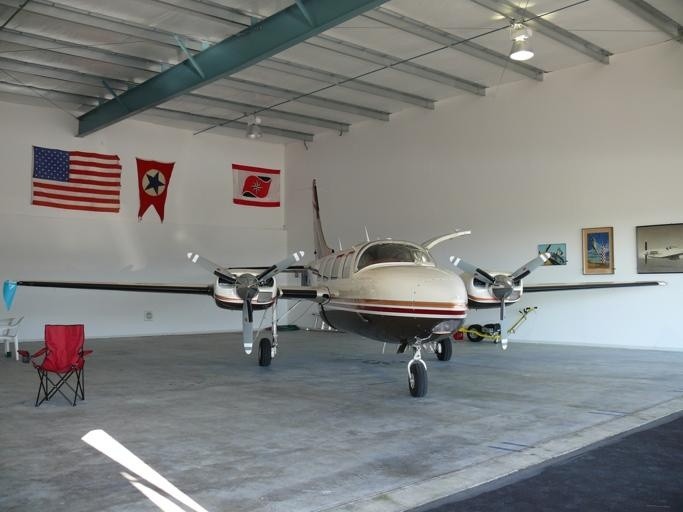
xmin=0 ymin=315 xmax=93 ymax=408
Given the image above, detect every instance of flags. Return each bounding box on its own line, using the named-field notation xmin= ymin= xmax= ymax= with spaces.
xmin=30 ymin=145 xmax=121 ymax=214
xmin=230 ymin=163 xmax=280 ymax=207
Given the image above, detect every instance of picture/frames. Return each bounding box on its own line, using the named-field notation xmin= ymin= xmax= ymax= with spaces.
xmin=583 ymin=223 xmax=683 ymax=275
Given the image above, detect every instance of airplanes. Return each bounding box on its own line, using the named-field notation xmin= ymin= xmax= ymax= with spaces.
xmin=641 ymin=239 xmax=683 ymax=265
xmin=16 ymin=174 xmax=670 ymax=403
